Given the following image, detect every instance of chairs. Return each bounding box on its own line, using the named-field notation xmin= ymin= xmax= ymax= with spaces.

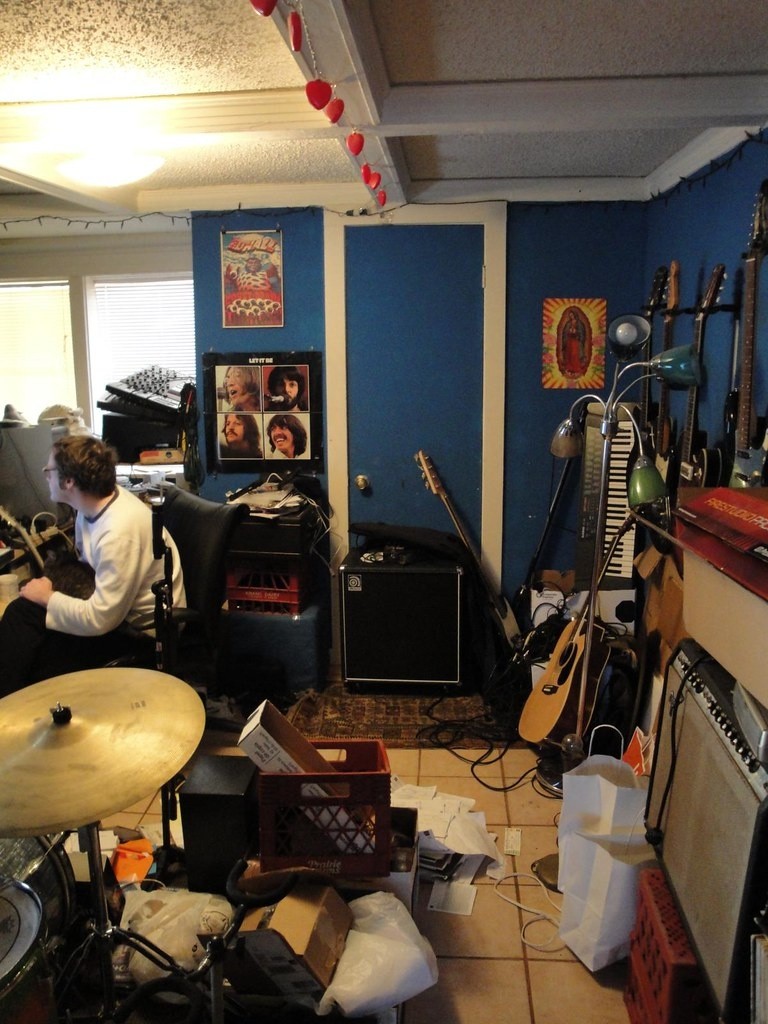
xmin=97 ymin=487 xmax=250 ymax=733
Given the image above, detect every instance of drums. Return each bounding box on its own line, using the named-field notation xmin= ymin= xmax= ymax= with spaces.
xmin=0 ymin=877 xmax=58 ymax=1023
xmin=1 ymin=834 xmax=80 ymax=948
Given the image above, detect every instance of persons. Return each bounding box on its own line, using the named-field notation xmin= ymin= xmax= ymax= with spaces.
xmin=217 ymin=366 xmax=307 ymax=459
xmin=0 ymin=435 xmax=187 ymax=702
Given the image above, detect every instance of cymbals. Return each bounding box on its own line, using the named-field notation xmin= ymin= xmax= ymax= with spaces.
xmin=0 ymin=665 xmax=207 ymax=841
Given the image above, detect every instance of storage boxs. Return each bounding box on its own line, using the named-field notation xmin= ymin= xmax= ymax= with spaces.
xmin=624 ymin=868 xmax=708 ymax=1024
xmin=339 ymin=548 xmax=465 ymax=694
xmin=258 ymin=739 xmax=391 ymax=878
xmin=334 ymin=806 xmax=417 ymax=914
xmin=227 ymin=566 xmax=300 ymax=617
xmin=197 ymin=885 xmax=356 ymax=994
xmin=236 ymin=699 xmax=375 ymax=854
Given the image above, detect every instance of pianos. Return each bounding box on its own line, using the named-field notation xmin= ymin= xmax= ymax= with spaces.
xmin=572 ymin=402 xmax=648 ymax=605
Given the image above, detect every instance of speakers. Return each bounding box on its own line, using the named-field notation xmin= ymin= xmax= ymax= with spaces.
xmin=643 ymin=637 xmax=768 ymax=1024
xmin=338 ymin=545 xmax=469 ymax=696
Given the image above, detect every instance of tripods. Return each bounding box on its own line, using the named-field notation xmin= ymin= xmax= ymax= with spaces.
xmin=52 ymin=820 xmax=247 ymax=1024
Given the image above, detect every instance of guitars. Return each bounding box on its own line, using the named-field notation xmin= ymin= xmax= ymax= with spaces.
xmin=729 ymin=226 xmax=768 ymax=491
xmin=648 ymin=260 xmax=677 ymax=513
xmin=630 ymin=264 xmax=669 ymax=491
xmin=677 ymin=264 xmax=726 ymax=499
xmin=412 ymin=448 xmax=544 ymax=689
xmin=517 ymin=505 xmax=646 ymax=749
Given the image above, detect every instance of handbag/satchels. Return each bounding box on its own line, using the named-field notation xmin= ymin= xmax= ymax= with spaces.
xmin=557 ymin=725 xmax=648 ymax=895
xmin=558 ymin=806 xmax=659 ymax=973
xmin=622 ymin=726 xmax=654 ymax=778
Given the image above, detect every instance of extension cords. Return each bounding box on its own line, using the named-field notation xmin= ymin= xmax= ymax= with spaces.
xmin=28 ymin=527 xmax=58 ymax=541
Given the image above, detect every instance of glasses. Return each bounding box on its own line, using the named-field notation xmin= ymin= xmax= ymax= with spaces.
xmin=42 ymin=465 xmax=58 ymax=474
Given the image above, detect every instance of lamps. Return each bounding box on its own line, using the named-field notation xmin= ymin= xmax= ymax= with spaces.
xmin=535 ymin=314 xmax=700 ymax=794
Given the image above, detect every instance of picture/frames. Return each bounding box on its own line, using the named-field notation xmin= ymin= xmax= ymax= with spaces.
xmin=221 ymin=228 xmax=284 ymax=329
xmin=202 ymin=352 xmax=324 ymax=475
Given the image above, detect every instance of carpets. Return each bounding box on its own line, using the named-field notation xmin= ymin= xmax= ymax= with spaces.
xmin=289 ymin=681 xmax=528 ymax=750
